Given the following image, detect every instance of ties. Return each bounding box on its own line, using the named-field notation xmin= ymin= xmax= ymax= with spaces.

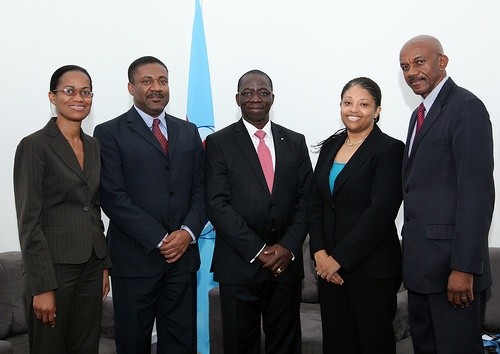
xmin=416 ymin=102 xmax=426 ymax=134
xmin=254 ymin=131 xmax=274 ymax=195
xmin=152 ymin=119 xmax=168 ymax=156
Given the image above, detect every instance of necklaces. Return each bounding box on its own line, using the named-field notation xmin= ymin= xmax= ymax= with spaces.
xmin=343 ymin=135 xmax=368 ymax=147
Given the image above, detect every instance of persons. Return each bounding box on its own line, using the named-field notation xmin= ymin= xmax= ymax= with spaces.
xmin=306 ymin=76 xmax=407 ymax=354
xmin=399 ymin=33 xmax=496 ymax=354
xmin=93 ymin=56 xmax=209 ymax=354
xmin=13 ymin=64 xmax=110 ymax=354
xmin=203 ymin=69 xmax=313 ymax=354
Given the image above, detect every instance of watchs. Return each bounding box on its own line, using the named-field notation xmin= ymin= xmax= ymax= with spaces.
xmin=290 ymin=250 xmax=297 ymax=263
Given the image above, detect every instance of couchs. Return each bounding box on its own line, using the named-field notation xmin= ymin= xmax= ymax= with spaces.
xmin=208 ymin=236 xmax=500 ymax=354
xmin=0 ymin=251 xmax=117 ymax=354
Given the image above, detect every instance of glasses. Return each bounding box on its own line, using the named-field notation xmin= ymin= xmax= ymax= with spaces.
xmin=53 ymin=86 xmax=93 ymax=98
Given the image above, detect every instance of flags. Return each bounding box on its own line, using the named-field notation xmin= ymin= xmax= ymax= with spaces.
xmin=183 ymin=0 xmax=220 ymax=354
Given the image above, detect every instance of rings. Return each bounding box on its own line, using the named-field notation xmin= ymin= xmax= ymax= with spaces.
xmin=277 ymin=267 xmax=281 ymax=275
xmin=461 ymin=297 xmax=468 ymax=300
xmin=317 ymin=271 xmax=322 ymax=277
